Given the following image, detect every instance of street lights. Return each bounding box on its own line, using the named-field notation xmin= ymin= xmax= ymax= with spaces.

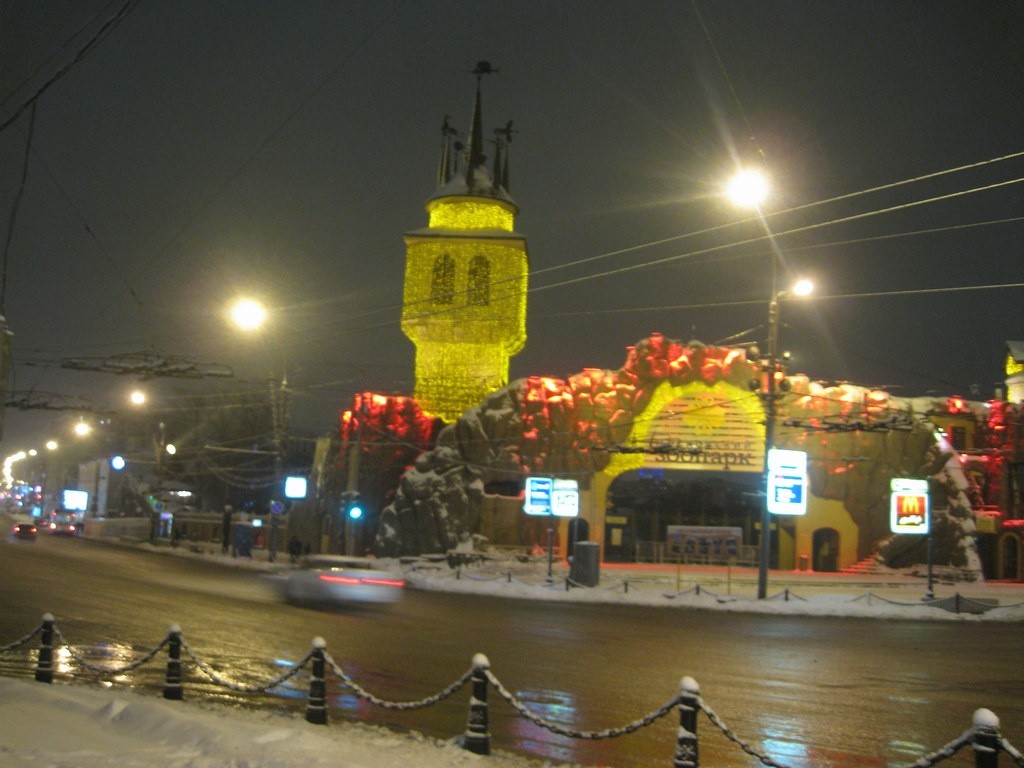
xmin=226 ymin=296 xmax=285 ymax=561
xmin=726 ymin=165 xmax=811 ymax=603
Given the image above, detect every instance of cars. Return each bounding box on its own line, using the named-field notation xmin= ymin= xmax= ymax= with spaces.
xmin=276 ymin=554 xmax=408 ymax=610
xmin=0 ymin=486 xmax=84 ymax=542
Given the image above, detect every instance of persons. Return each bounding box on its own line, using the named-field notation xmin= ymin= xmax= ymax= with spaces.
xmin=287 ymin=537 xmax=302 ymax=561
xmin=80 ymin=523 xmax=84 ymax=532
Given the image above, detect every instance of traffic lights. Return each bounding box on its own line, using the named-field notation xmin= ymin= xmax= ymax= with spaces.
xmin=347 ymin=505 xmax=367 ymax=523
